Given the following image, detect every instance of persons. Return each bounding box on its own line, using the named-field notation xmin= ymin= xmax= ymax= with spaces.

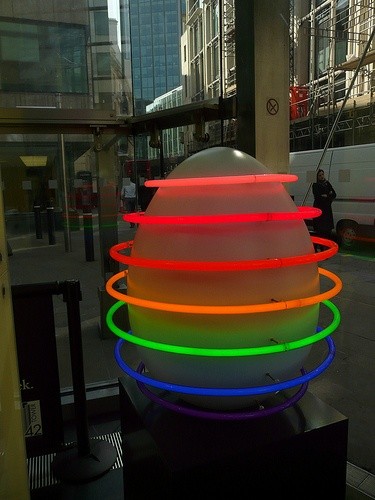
xmin=121 ymin=176 xmax=138 ymax=228
xmin=138 ymin=176 xmax=156 ymax=212
xmin=312 ymin=169 xmax=337 ymax=260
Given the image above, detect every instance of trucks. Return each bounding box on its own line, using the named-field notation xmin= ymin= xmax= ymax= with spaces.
xmin=289 ymin=145 xmax=375 ymax=251
